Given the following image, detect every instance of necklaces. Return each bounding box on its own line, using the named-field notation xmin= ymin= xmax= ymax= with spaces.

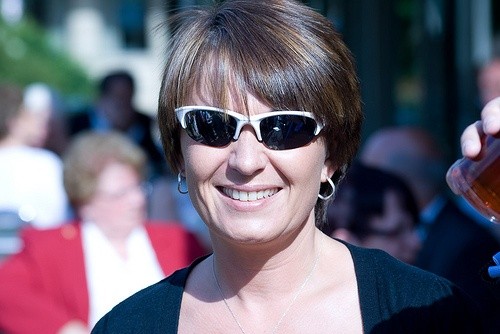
xmin=212 ymin=243 xmax=321 ymax=334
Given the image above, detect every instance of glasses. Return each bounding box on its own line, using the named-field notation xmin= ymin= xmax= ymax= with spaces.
xmin=174 ymin=105 xmax=325 ymax=151
xmin=98 ymin=181 xmax=140 ymax=204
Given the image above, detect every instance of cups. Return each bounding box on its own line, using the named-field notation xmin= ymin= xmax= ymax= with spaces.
xmin=445 ymin=132 xmax=500 ymax=225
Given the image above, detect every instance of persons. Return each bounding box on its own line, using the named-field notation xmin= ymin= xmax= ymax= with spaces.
xmin=0 ymin=83 xmax=69 ymax=249
xmin=0 ymin=131 xmax=211 ymax=334
xmin=360 ymin=126 xmax=445 ymax=241
xmin=93 ymin=0 xmax=477 ymax=334
xmin=64 ymin=72 xmax=169 ymax=178
xmin=325 ymin=167 xmax=423 ymax=264
xmin=461 ymin=94 xmax=499 ymax=283
